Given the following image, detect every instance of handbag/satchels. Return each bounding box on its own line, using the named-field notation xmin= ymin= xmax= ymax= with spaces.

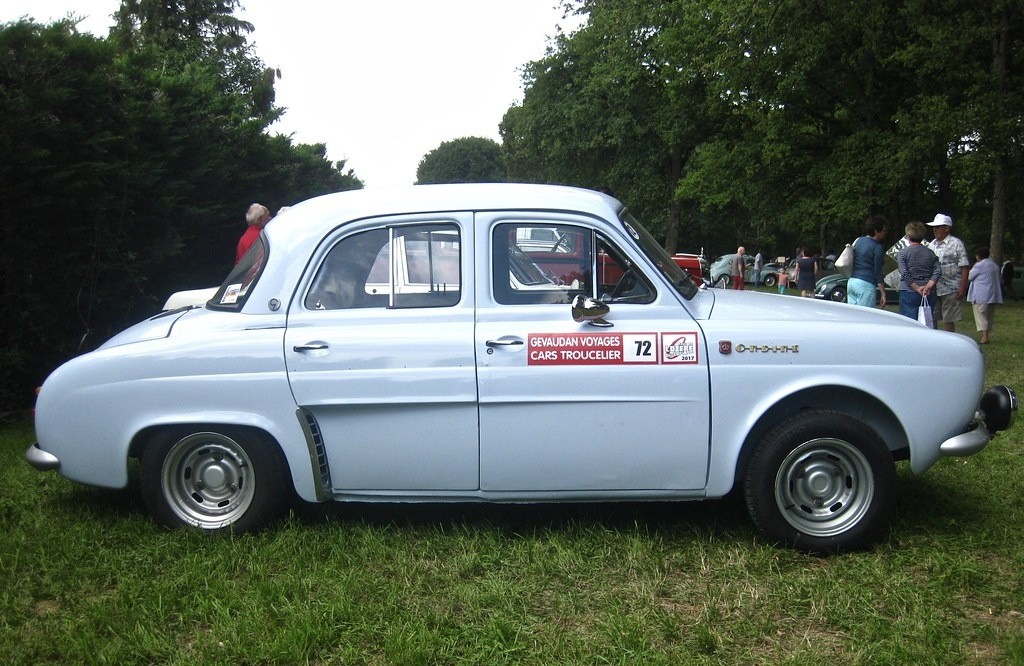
xmin=835 ymin=244 xmax=855 ymax=279
xmin=917 ymin=294 xmax=934 ymax=329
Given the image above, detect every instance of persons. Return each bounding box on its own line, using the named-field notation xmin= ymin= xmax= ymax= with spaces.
xmin=733 ymin=247 xmax=750 ymax=290
xmin=754 ymin=249 xmax=762 ymax=288
xmin=1000 ymin=254 xmax=1018 ymax=300
xmin=846 ymin=216 xmax=888 ymax=309
xmin=898 ymin=221 xmax=941 ymax=329
xmin=926 ymin=214 xmax=968 ymax=332
xmin=774 ymin=268 xmax=789 ymax=295
xmin=794 ymin=248 xmax=818 ymax=298
xmin=233 ymin=203 xmax=272 ymax=288
xmin=826 ymin=250 xmax=837 ymax=270
xmin=968 ymin=248 xmax=1003 ymax=344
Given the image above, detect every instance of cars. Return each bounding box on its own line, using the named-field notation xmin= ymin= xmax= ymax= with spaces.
xmin=159 ymin=229 xmax=725 ymax=312
xmin=711 ymin=253 xmax=898 ymax=304
xmin=25 ymin=183 xmax=1018 ymax=556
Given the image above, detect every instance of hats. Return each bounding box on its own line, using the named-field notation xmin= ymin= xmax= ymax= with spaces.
xmin=926 ymin=214 xmax=952 ymax=227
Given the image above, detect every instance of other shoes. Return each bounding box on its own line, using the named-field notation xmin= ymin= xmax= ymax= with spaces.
xmin=977 ymin=340 xmax=990 ymax=344
